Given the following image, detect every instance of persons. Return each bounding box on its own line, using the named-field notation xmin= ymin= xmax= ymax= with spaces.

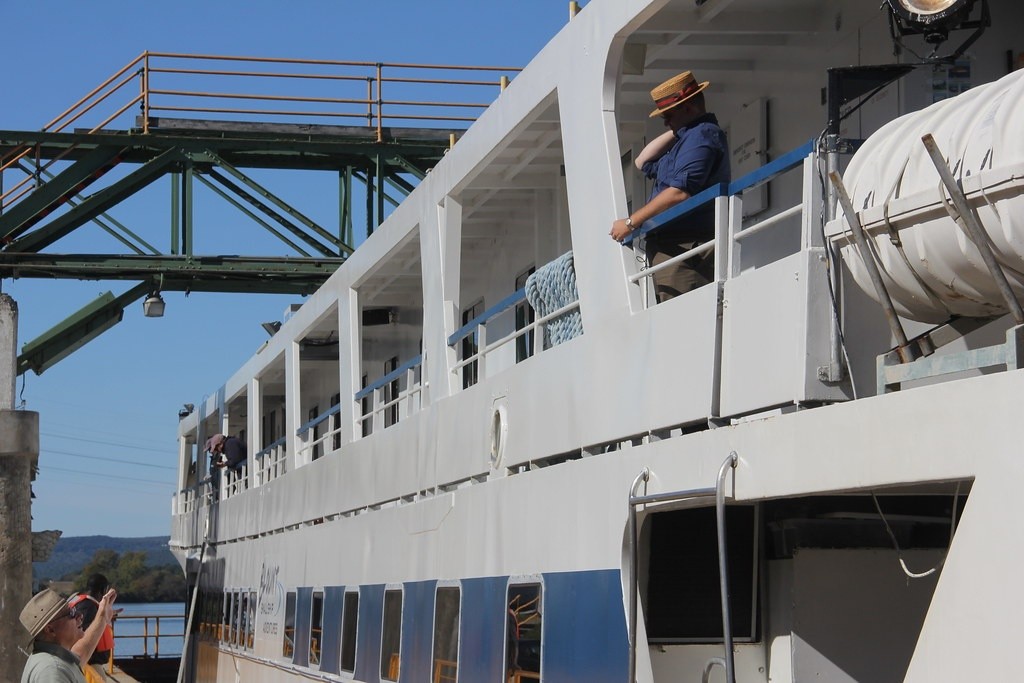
xmin=203 ymin=432 xmax=248 ymax=497
xmin=39 ymin=573 xmax=115 ymax=683
xmin=609 ymin=70 xmax=731 ymax=305
xmin=19 ymin=589 xmax=117 ymax=683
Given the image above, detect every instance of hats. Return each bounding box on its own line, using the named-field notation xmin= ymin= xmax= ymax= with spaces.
xmin=210 ymin=434 xmax=223 ymax=452
xmin=19 ymin=588 xmax=79 ymax=650
xmin=649 ymin=70 xmax=710 ymax=118
xmin=203 ymin=438 xmax=212 ymax=452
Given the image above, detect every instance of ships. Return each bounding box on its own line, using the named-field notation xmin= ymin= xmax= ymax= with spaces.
xmin=164 ymin=0 xmax=1024 ymax=682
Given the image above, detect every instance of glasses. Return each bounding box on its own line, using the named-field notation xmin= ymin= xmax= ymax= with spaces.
xmin=660 ymin=107 xmax=679 ymax=120
xmin=48 ymin=606 xmax=77 ymax=624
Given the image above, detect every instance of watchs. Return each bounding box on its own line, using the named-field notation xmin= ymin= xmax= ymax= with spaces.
xmin=624 ymin=218 xmax=636 ymax=231
